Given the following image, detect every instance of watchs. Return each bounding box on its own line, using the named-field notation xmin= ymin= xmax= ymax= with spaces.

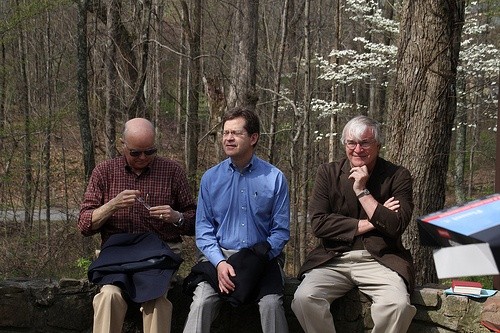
xmin=357 ymin=188 xmax=370 ymax=199
xmin=173 ymin=211 xmax=184 ymax=227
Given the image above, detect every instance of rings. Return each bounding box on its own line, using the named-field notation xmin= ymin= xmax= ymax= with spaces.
xmin=159 ymin=214 xmax=163 ymax=220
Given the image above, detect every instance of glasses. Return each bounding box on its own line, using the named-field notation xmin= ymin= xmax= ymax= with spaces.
xmin=125 ymin=142 xmax=157 ymax=157
xmin=345 ymin=140 xmax=376 ymax=149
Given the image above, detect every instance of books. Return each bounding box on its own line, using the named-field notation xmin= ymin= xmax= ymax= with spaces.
xmin=451 ymin=281 xmax=482 ymax=295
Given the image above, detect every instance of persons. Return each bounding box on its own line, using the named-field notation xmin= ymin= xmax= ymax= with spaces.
xmin=289 ymin=115 xmax=416 ymax=333
xmin=78 ymin=117 xmax=199 ymax=333
xmin=181 ymin=107 xmax=294 ymax=333
